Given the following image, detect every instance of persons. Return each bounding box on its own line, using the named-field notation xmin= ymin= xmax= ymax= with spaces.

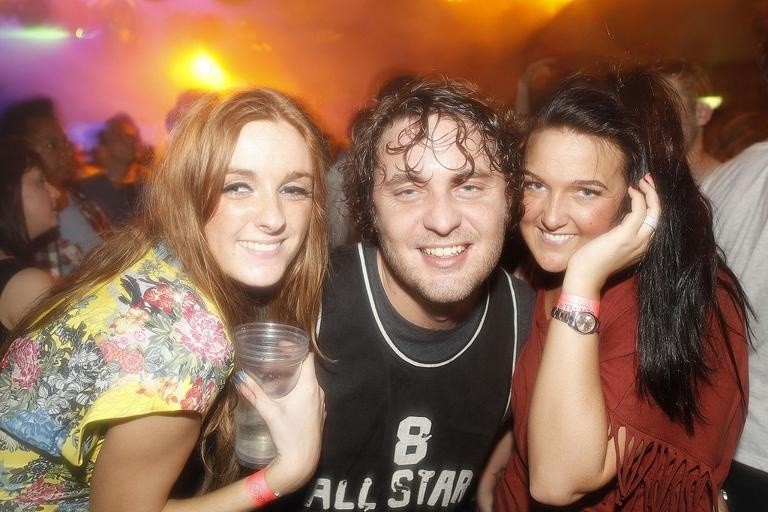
xmin=2 ymin=56 xmax=767 ymax=511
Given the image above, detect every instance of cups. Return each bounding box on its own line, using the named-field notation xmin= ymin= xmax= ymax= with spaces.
xmin=229 ymin=320 xmax=312 ymax=474
